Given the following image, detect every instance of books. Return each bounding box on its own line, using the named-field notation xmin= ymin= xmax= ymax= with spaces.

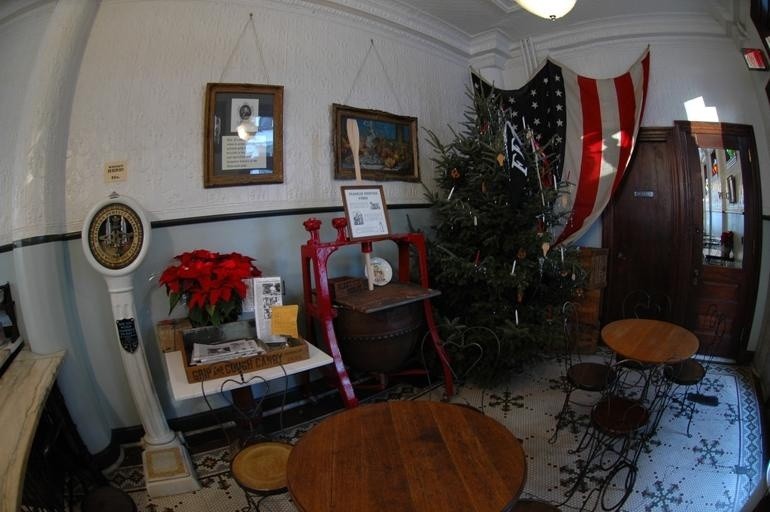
xmin=238 ymin=277 xmax=286 ymax=344
xmin=188 ymin=340 xmax=264 ymax=368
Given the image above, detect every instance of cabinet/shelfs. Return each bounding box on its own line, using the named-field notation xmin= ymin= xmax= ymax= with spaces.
xmin=688 ymin=279 xmax=760 ymax=359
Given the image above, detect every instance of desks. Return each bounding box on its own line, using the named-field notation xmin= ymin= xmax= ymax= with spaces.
xmin=164 ymin=339 xmax=334 ymax=450
xmin=286 ymin=399 xmax=527 ymax=512
xmin=565 ymin=319 xmax=700 ymax=456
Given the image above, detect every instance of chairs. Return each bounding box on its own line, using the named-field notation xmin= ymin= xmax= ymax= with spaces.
xmin=612 ymin=288 xmax=677 ymax=412
xmin=644 ymin=304 xmax=729 ymax=439
xmin=420 ymin=320 xmax=501 ymax=414
xmin=203 ymin=354 xmax=295 ymax=512
xmin=547 ymin=302 xmax=620 ymax=447
xmin=550 ymin=358 xmax=677 ymax=512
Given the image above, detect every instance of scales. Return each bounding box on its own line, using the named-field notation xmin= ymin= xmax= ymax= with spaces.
xmin=81 ymin=195 xmax=200 ymax=499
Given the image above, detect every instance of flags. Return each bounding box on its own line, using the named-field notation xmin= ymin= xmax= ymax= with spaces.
xmin=461 ymin=44 xmax=652 ymax=253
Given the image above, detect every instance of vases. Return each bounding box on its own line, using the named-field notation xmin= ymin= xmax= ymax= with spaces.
xmin=184 ymin=293 xmax=241 ymax=327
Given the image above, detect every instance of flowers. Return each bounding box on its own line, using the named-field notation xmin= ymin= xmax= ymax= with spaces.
xmin=158 ymin=247 xmax=263 ymax=327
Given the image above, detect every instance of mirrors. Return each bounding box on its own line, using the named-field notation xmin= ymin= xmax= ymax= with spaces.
xmin=687 ymin=132 xmax=751 ymax=284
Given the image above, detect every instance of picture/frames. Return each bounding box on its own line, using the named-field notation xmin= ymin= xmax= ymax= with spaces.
xmin=727 ymin=175 xmax=736 ymax=203
xmin=340 ymin=185 xmax=392 ymax=241
xmin=332 ymin=100 xmax=422 ymax=183
xmin=201 ymin=82 xmax=282 ymax=187
